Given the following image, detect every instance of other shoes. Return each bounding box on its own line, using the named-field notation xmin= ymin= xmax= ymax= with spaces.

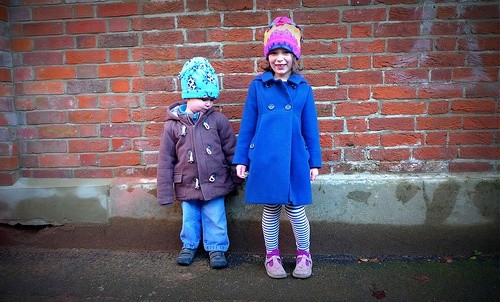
xmin=292 ymin=254 xmax=312 ymax=278
xmin=264 ymin=255 xmax=287 ymax=278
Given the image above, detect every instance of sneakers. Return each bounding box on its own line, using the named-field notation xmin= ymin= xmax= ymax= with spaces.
xmin=177 ymin=249 xmax=196 ymax=264
xmin=209 ymin=251 xmax=227 ymax=267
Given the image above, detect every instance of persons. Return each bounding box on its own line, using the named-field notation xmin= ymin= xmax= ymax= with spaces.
xmin=232 ymin=16 xmax=322 ymax=279
xmin=156 ymin=57 xmax=245 ymax=268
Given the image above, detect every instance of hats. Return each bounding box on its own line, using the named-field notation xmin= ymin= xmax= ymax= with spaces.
xmin=178 ymin=57 xmax=219 ymax=99
xmin=264 ymin=16 xmax=303 ymax=60
